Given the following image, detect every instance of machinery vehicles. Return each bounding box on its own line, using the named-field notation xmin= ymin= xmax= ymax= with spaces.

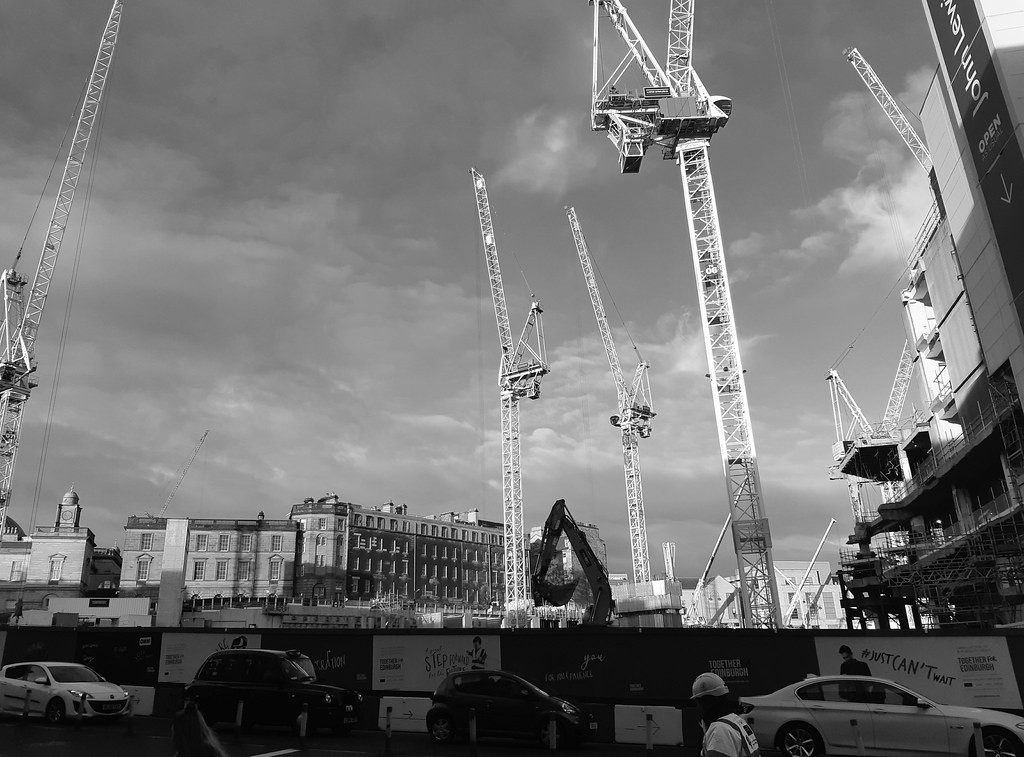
xmin=532 ymin=497 xmax=617 ymax=626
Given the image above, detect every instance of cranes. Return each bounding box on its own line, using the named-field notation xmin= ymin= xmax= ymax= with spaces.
xmin=843 ymin=47 xmax=932 ymax=174
xmin=464 ymin=168 xmax=549 ymax=627
xmin=0 ymin=1 xmax=127 ymax=630
xmin=591 ymin=0 xmax=787 ymax=627
xmin=823 ymin=340 xmax=917 ymax=565
xmin=142 ymin=431 xmax=210 ymax=518
xmin=564 ymin=199 xmax=837 ymax=627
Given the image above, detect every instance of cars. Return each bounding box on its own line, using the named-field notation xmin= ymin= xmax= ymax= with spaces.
xmin=426 ymin=670 xmax=598 ymax=750
xmin=184 ymin=648 xmax=367 ymax=738
xmin=0 ymin=662 xmax=131 ymax=727
xmin=737 ymin=675 xmax=1024 ymax=757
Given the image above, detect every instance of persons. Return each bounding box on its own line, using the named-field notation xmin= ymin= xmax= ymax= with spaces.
xmin=171 ymin=703 xmax=230 ymax=757
xmin=689 ymin=673 xmax=762 ymax=757
xmin=871 ymin=685 xmax=887 ymax=704
xmin=466 ymin=637 xmax=488 ymax=670
xmin=838 ymin=645 xmax=873 ymax=676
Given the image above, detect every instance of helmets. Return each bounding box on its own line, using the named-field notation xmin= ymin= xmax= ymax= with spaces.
xmin=690 ymin=673 xmax=729 ymax=697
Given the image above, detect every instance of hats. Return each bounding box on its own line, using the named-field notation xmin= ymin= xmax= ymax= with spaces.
xmin=229 ymin=636 xmax=248 ymax=649
